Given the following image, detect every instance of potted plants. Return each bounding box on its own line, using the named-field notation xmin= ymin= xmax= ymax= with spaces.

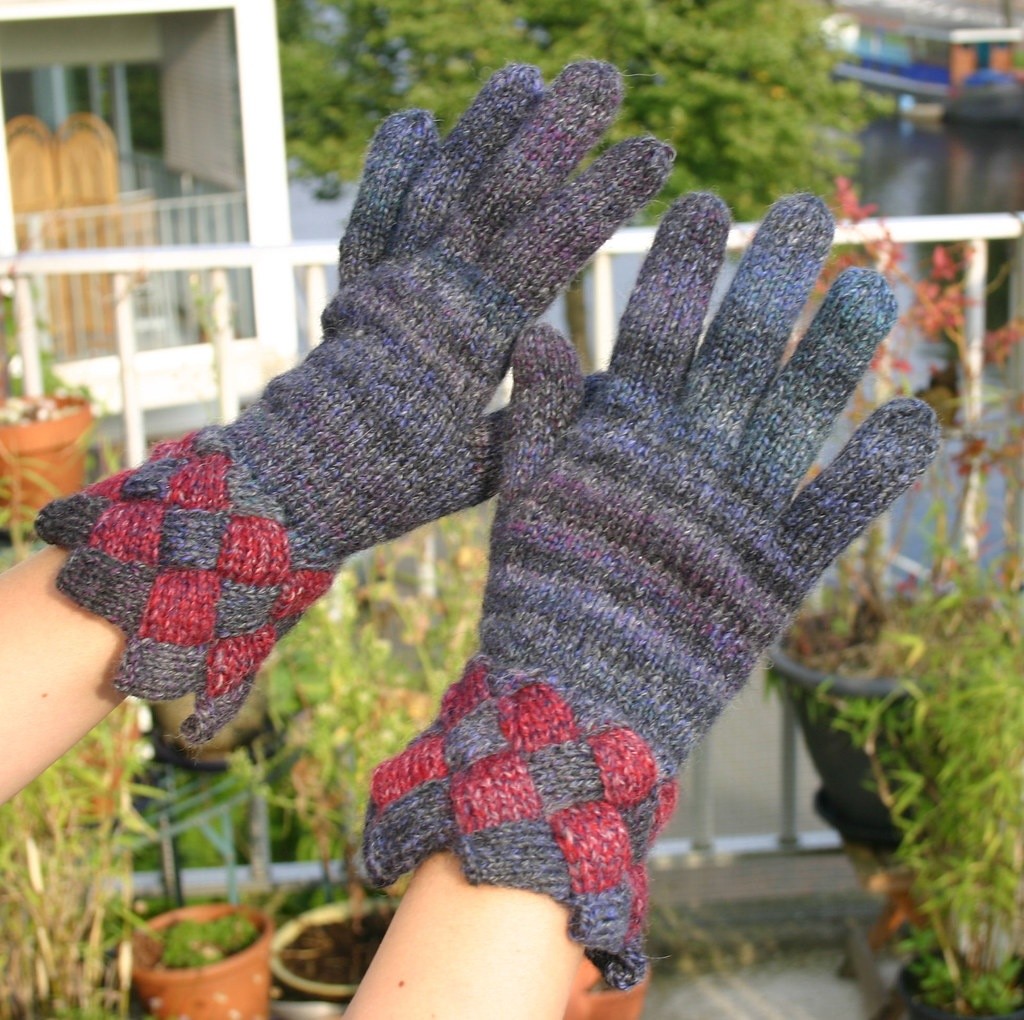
xmin=269 ymin=570 xmax=452 ymax=1002
xmin=765 ymin=175 xmax=1024 ymax=849
xmin=116 ymin=905 xmax=275 ymax=1020
xmin=814 ymin=496 xmax=1024 ymax=1020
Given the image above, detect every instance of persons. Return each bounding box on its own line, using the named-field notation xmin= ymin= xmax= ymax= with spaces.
xmin=0 ymin=61 xmax=941 ymax=1020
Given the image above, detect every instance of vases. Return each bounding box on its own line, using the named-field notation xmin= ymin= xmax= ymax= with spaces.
xmin=1 ymin=392 xmax=92 ymax=526
xmin=560 ymin=963 xmax=651 ymax=1019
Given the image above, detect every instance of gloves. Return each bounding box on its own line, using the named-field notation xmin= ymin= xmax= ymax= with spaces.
xmin=362 ymin=195 xmax=942 ymax=984
xmin=35 ymin=58 xmax=677 ymax=747
